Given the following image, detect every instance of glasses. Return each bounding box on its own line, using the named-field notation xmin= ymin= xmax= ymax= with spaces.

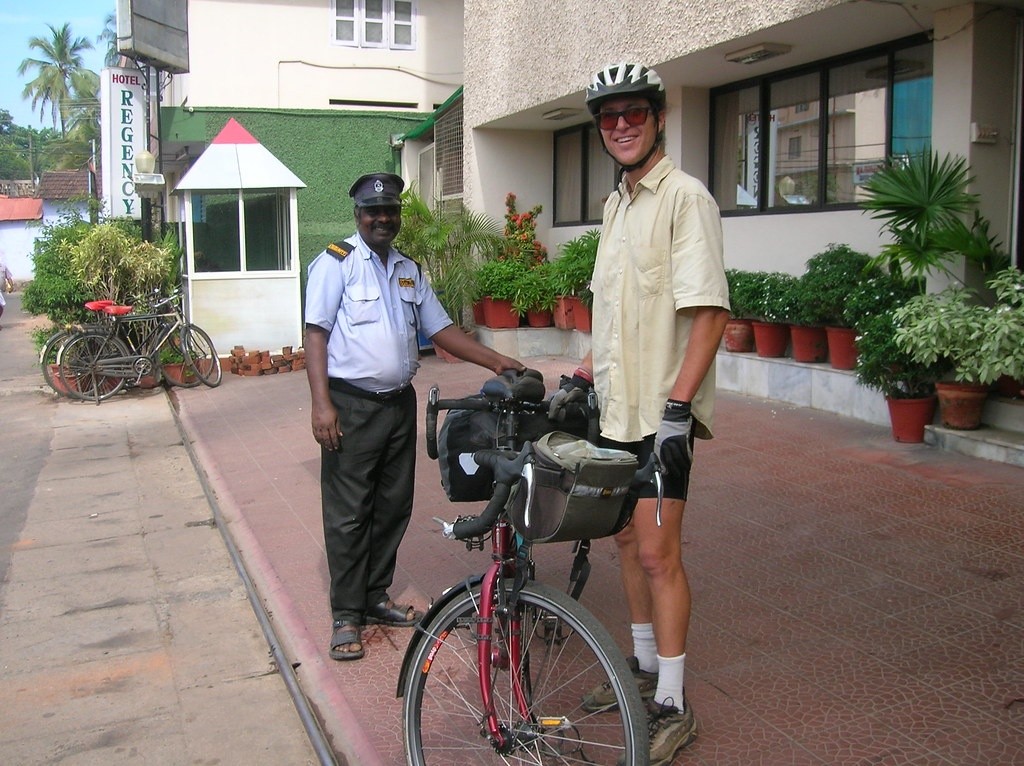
xmin=594 ymin=107 xmax=651 ymax=130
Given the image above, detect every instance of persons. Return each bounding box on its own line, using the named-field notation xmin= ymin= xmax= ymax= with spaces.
xmin=558 ymin=62 xmax=733 ymax=766
xmin=303 ymin=173 xmax=527 ymax=662
xmin=0 ymin=262 xmax=14 ymax=331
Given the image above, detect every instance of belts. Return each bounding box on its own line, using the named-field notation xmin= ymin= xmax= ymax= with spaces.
xmin=329 ymin=381 xmax=411 ymax=404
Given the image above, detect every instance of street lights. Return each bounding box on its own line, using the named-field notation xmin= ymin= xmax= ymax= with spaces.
xmin=134 ymin=150 xmax=166 ymax=243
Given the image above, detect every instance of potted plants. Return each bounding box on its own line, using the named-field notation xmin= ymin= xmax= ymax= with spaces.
xmin=722 ymin=270 xmax=759 ymax=353
xmin=767 ymin=273 xmax=827 ymax=363
xmin=987 ymin=266 xmax=1024 ymax=401
xmin=795 ymin=245 xmax=890 ymax=372
xmin=20 ymin=192 xmax=200 ymax=394
xmin=733 ymin=272 xmax=795 ymax=358
xmin=393 ymin=181 xmax=600 ymax=364
xmin=855 ymin=310 xmax=936 ymax=443
xmin=892 ymin=268 xmax=993 ymax=428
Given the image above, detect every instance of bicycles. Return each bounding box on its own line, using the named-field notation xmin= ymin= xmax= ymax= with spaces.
xmin=395 ymin=368 xmax=673 ymax=766
xmin=37 ymin=280 xmax=223 ymax=405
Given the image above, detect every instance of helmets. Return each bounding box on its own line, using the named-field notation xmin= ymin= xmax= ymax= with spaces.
xmin=584 ymin=63 xmax=666 ymax=119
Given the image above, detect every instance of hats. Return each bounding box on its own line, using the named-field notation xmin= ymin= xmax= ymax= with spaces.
xmin=349 ymin=173 xmax=405 ymax=208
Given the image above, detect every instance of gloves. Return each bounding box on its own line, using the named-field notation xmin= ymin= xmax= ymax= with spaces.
xmin=653 ymin=399 xmax=693 ymax=488
xmin=547 ymin=374 xmax=594 ymax=424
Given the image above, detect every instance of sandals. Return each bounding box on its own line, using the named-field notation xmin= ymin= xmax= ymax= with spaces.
xmin=361 ymin=600 xmax=423 ymax=627
xmin=329 ymin=620 xmax=365 ymax=661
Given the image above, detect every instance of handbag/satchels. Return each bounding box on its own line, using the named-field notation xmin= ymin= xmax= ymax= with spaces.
xmin=439 ymin=393 xmax=601 ymax=502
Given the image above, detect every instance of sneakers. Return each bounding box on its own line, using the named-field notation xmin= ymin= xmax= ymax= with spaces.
xmin=616 ymin=686 xmax=698 ymax=766
xmin=580 ymin=655 xmax=659 ymax=713
